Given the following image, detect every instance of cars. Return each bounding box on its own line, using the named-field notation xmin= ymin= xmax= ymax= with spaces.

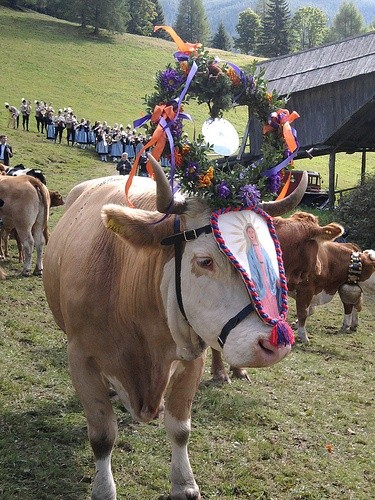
xmin=277 ymin=170 xmax=336 ymax=208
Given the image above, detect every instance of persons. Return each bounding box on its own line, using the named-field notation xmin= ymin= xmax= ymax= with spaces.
xmin=140 ymin=156 xmax=149 ymax=177
xmin=5 ymin=105 xmax=20 ymax=129
xmin=182 ymin=134 xmax=190 ymax=143
xmin=35 ymin=101 xmax=170 ymax=167
xmin=117 ymin=152 xmax=132 ymax=175
xmin=21 ymin=100 xmax=31 ymax=131
xmin=0 ymin=135 xmax=13 ymax=166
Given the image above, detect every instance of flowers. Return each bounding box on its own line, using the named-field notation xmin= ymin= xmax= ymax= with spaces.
xmin=124 ymin=25 xmax=300 ymax=208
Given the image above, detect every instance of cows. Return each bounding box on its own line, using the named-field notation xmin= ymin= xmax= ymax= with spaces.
xmin=288 ymin=242 xmax=375 ymax=345
xmin=42 ymin=153 xmax=307 ymax=500
xmin=0 ymin=164 xmax=65 ymax=275
xmin=211 ymin=210 xmax=343 ymax=383
